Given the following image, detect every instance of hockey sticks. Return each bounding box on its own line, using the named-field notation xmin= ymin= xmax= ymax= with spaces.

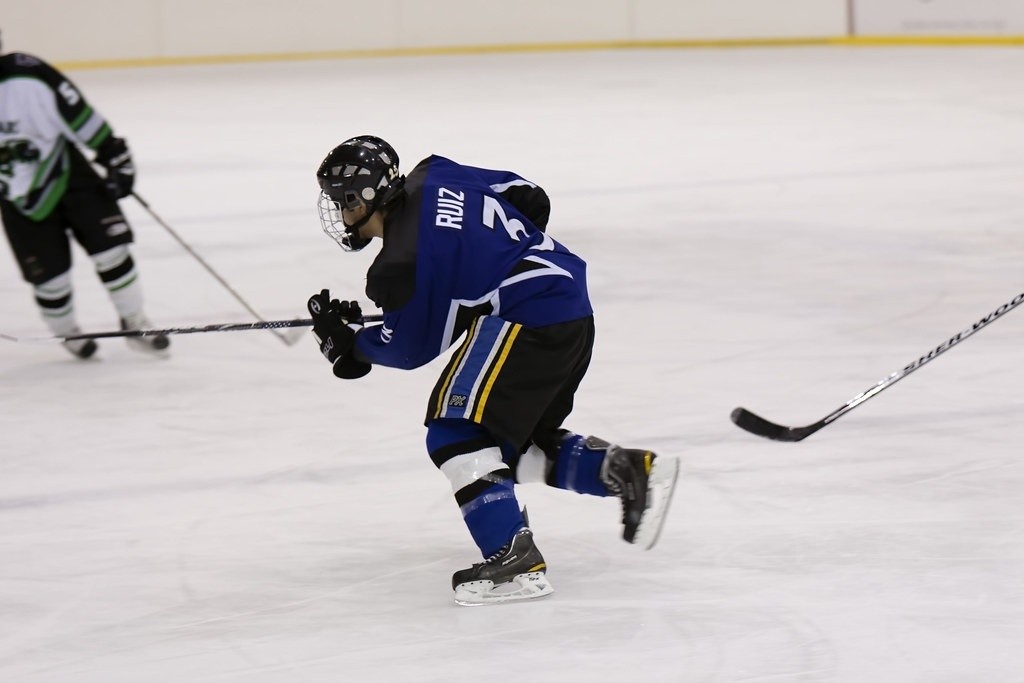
xmin=0 ymin=313 xmax=385 ymax=344
xmin=130 ymin=190 xmax=312 ymax=345
xmin=730 ymin=294 xmax=1024 ymax=444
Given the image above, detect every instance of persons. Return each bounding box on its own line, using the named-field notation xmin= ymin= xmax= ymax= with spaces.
xmin=307 ymin=134 xmax=658 ymax=591
xmin=0 ymin=28 xmax=170 ymax=359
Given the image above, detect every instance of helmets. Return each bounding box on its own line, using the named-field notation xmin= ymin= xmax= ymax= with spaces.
xmin=316 ymin=134 xmax=399 ymax=252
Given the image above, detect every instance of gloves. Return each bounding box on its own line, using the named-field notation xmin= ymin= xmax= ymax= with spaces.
xmin=93 ymin=137 xmax=135 ymax=198
xmin=307 ymin=289 xmax=372 ymax=379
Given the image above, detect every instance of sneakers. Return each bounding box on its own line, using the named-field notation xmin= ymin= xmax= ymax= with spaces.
xmin=120 ymin=314 xmax=169 ymax=357
xmin=56 ymin=328 xmax=98 ymax=359
xmin=452 ymin=503 xmax=554 ymax=605
xmin=587 ymin=437 xmax=679 ymax=550
